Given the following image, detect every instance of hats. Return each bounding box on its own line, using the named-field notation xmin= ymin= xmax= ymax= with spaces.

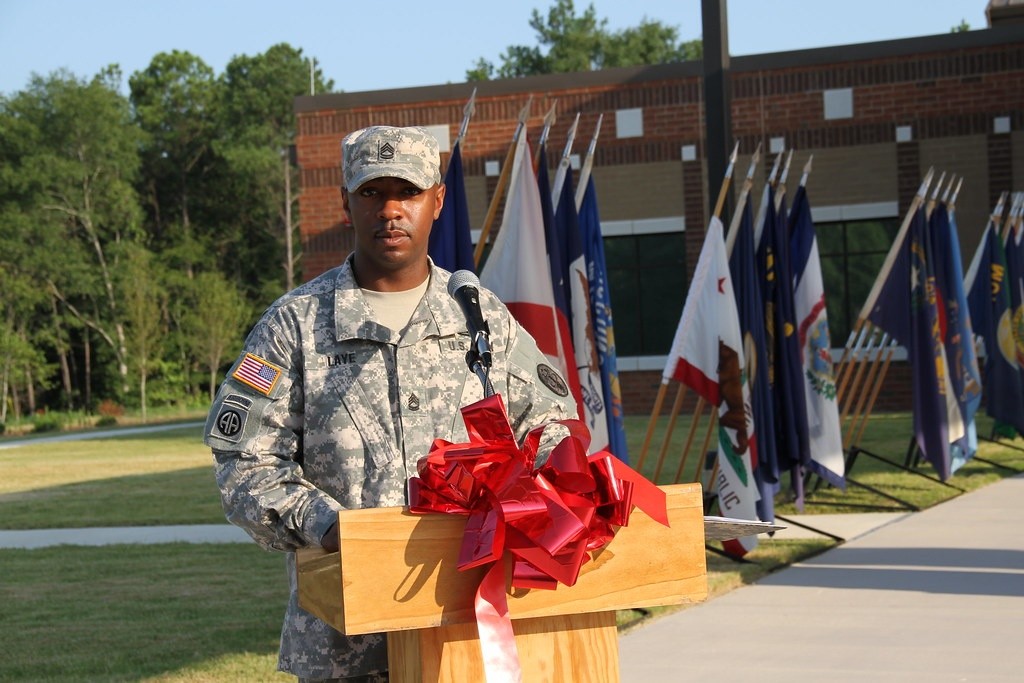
xmin=341 ymin=126 xmax=441 ymax=193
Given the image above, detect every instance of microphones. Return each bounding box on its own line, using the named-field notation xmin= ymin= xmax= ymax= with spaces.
xmin=446 ymin=270 xmax=492 ymax=367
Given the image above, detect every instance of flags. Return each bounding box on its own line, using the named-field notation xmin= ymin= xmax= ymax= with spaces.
xmin=476 ymin=123 xmax=630 ymax=465
xmin=428 ymin=140 xmax=476 ymax=275
xmin=868 ymin=204 xmax=982 ymax=483
xmin=967 ymin=218 xmax=1024 ymax=438
xmin=674 ymin=187 xmax=848 ymax=558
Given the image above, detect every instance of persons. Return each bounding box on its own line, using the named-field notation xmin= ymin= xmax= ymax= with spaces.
xmin=204 ymin=125 xmax=581 ymax=683
